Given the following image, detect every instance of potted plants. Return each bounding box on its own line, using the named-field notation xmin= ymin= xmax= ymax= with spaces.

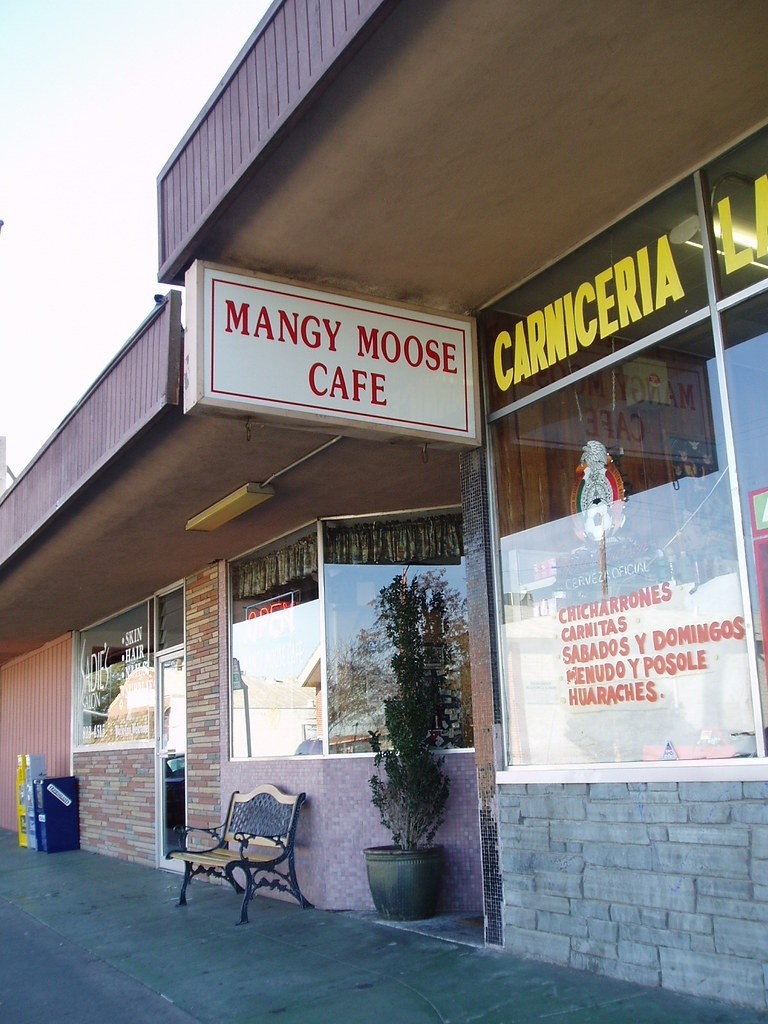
xmin=362 ymin=576 xmax=463 ymax=921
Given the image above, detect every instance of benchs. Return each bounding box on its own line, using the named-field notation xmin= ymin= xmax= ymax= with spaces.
xmin=165 ymin=784 xmax=315 ymax=926
